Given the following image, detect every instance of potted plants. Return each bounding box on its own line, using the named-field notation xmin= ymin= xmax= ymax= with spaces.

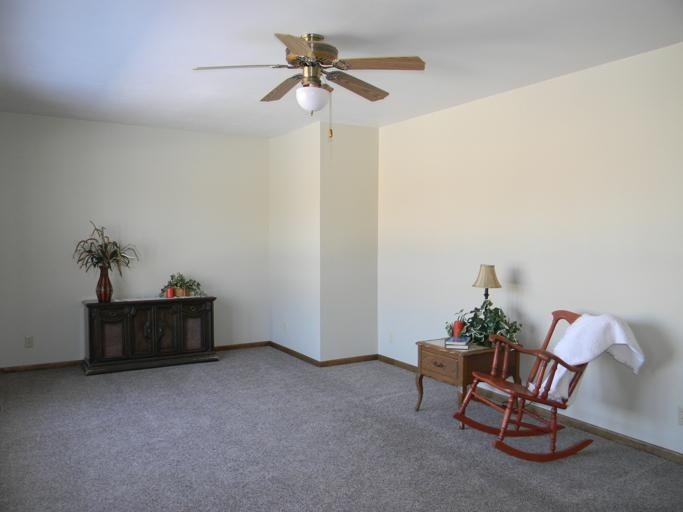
xmin=72 ymin=221 xmax=138 ymax=302
xmin=158 ymin=271 xmax=207 ymax=298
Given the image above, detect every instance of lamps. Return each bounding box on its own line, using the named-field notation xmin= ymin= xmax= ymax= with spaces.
xmin=295 ymin=87 xmax=330 ymax=117
xmin=471 ymin=263 xmax=502 ymax=301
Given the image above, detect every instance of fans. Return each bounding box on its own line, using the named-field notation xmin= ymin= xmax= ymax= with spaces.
xmin=190 ymin=33 xmax=426 ymax=138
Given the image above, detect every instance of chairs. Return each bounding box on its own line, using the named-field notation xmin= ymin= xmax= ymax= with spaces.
xmin=453 ymin=309 xmax=593 ymax=461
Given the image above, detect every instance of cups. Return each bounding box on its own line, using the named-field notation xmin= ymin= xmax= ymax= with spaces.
xmin=454 ymin=321 xmax=465 ymax=337
xmin=167 ymin=287 xmax=174 ymax=298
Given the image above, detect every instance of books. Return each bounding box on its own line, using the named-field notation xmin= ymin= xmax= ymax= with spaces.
xmin=444 ymin=335 xmax=471 ymax=350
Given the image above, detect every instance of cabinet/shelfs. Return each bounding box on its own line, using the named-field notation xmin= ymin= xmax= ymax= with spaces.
xmin=81 ymin=295 xmax=218 ymax=375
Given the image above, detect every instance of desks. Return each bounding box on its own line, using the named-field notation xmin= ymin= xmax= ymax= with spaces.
xmin=415 ymin=337 xmax=523 ymax=430
